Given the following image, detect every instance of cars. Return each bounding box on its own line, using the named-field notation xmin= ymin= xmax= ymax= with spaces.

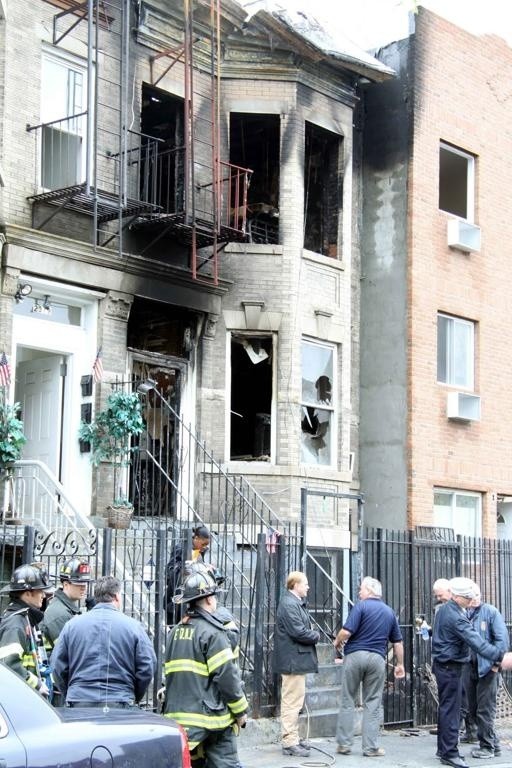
xmin=0 ymin=662 xmax=192 ymax=768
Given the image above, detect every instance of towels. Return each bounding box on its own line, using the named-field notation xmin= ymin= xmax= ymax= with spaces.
xmin=265 ymin=526 xmax=280 ymax=554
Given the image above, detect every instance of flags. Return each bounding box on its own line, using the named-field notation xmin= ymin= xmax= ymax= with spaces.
xmin=93 ymin=346 xmax=105 ymax=384
xmin=0 ymin=354 xmax=13 ymax=391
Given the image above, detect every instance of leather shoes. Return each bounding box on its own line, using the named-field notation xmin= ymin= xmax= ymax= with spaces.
xmin=458 ymin=729 xmax=465 ymax=734
xmin=440 ymin=756 xmax=470 ymax=768
xmin=436 ymin=751 xmax=464 ymax=761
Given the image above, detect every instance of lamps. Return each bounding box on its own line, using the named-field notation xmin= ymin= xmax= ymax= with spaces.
xmin=28 ymin=296 xmax=41 ymax=314
xmin=41 ymin=296 xmax=53 ymax=316
xmin=15 ymin=282 xmax=32 ymax=303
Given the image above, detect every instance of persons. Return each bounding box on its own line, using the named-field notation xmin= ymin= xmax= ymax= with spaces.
xmin=464 ymin=583 xmax=510 ymax=758
xmin=432 ymin=577 xmax=512 ymax=768
xmin=275 ymin=570 xmax=321 ymax=758
xmin=1 ymin=562 xmax=50 ymax=704
xmin=332 ymin=576 xmax=405 ymax=757
xmin=38 ymin=558 xmax=95 ymax=707
xmin=414 ymin=577 xmax=479 ymax=744
xmin=49 ymin=576 xmax=158 ymax=711
xmin=163 ymin=525 xmax=213 ymax=630
xmin=175 ymin=562 xmax=248 ymax=768
xmin=160 ymin=570 xmax=251 ymax=768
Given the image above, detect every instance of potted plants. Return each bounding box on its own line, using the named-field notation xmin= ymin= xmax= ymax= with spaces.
xmin=0 ymin=401 xmax=27 ymax=547
xmin=79 ymin=393 xmax=146 ymax=530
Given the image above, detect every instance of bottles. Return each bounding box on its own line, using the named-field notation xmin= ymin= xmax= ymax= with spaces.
xmin=421 ymin=620 xmax=431 ymax=641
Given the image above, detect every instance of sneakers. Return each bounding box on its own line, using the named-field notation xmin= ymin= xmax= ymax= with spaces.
xmin=283 ymin=746 xmax=310 ymax=757
xmin=363 ymin=747 xmax=385 ymax=756
xmin=299 ymin=741 xmax=310 ymax=750
xmin=338 ymin=745 xmax=351 ymax=755
xmin=471 ymin=747 xmax=494 ymax=759
xmin=429 ymin=728 xmax=438 ymax=735
xmin=459 ymin=736 xmax=479 ymax=743
xmin=495 ymin=749 xmax=501 ymax=757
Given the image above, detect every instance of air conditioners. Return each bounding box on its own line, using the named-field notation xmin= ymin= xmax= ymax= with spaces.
xmin=447 ymin=219 xmax=483 ymax=256
xmin=446 ymin=392 xmax=482 ymax=424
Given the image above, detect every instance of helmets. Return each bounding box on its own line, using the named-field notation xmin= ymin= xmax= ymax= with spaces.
xmin=171 ymin=572 xmax=228 ymax=604
xmin=60 ymin=558 xmax=94 ymax=585
xmin=0 ymin=562 xmax=52 ymax=593
xmin=177 ymin=562 xmax=226 ymax=591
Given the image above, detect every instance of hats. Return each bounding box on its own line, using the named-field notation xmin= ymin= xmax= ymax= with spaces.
xmin=449 ymin=578 xmax=476 ymax=599
